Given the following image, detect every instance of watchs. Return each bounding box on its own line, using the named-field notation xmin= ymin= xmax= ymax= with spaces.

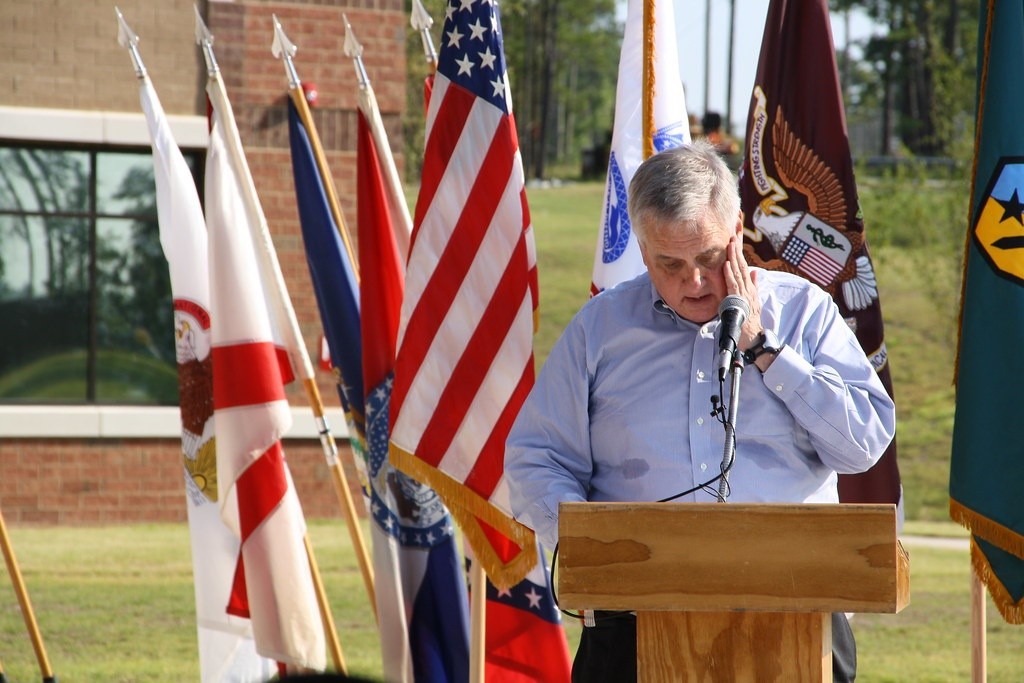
xmin=744 ymin=329 xmax=782 ymax=363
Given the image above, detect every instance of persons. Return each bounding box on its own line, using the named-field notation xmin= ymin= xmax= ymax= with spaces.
xmin=688 ymin=110 xmax=744 ymax=173
xmin=504 ymin=136 xmax=898 ymax=683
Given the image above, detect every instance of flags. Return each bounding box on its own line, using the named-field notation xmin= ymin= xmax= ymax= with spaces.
xmin=738 ymin=0 xmax=902 ymax=509
xmin=388 ymin=0 xmax=537 ymax=590
xmin=949 ymin=0 xmax=1024 ymax=627
xmin=204 ymin=72 xmax=328 ymax=677
xmin=349 ymin=88 xmax=473 ymax=683
xmin=423 ymin=60 xmax=574 ymax=683
xmin=576 ymin=0 xmax=703 ymax=626
xmin=284 ymin=93 xmax=373 ymax=510
xmin=140 ymin=84 xmax=307 ymax=683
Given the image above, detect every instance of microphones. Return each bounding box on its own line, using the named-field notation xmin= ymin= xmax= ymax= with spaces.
xmin=718 ymin=295 xmax=752 ymax=380
xmin=711 ymin=395 xmax=720 ymax=409
